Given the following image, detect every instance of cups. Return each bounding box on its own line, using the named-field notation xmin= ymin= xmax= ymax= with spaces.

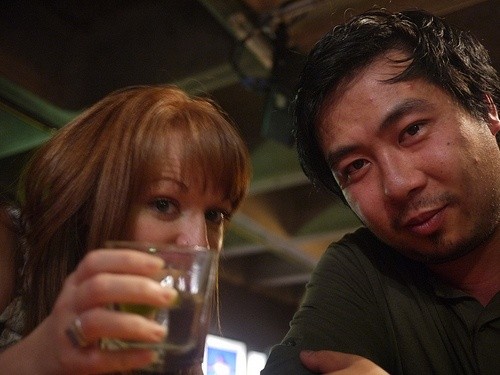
xmin=103 ymin=240 xmax=220 ymax=354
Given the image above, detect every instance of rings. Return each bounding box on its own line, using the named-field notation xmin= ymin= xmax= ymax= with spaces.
xmin=66 ymin=318 xmax=86 ymax=350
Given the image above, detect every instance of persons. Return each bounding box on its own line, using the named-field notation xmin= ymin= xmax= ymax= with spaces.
xmin=0 ymin=83 xmax=252 ymax=375
xmin=259 ymin=7 xmax=500 ymax=375
